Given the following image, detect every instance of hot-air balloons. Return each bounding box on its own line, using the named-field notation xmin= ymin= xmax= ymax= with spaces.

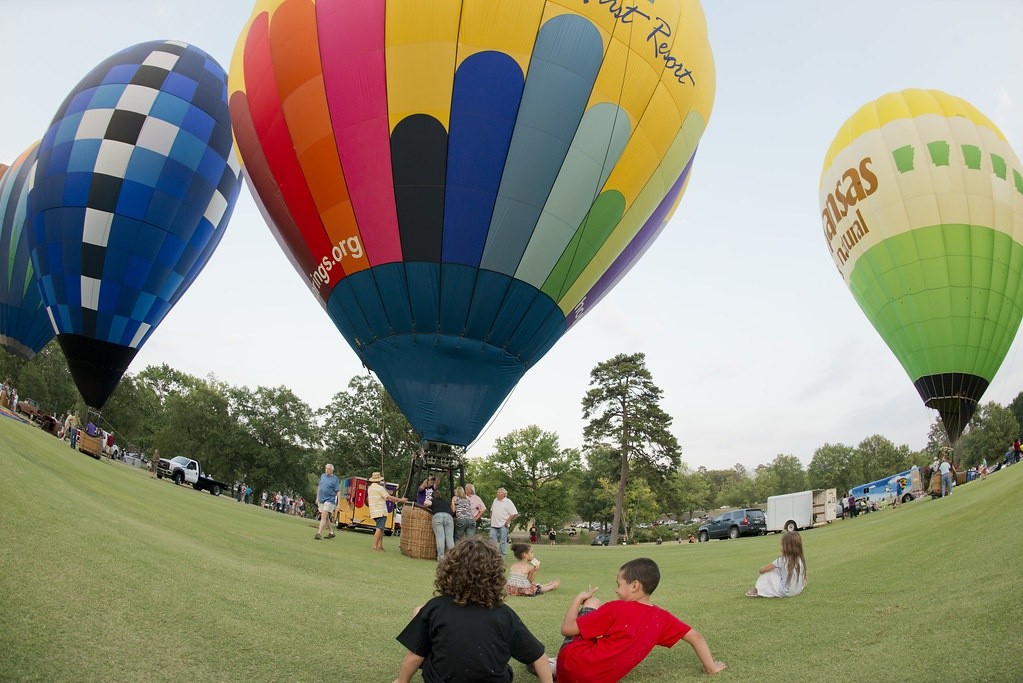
xmin=0 ymin=138 xmax=57 ymax=409
xmin=227 ymin=0 xmax=719 ymax=562
xmin=819 ymin=84 xmax=1023 ymax=499
xmin=26 ymin=38 xmax=243 ymax=462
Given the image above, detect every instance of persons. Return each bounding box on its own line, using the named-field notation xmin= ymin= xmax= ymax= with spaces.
xmin=549 ymin=526 xmax=556 ymax=545
xmin=528 ymin=558 xmax=727 ymax=683
xmin=393 ymin=535 xmax=555 ymax=683
xmin=149 ymin=448 xmax=160 ymax=479
xmin=201 ymin=472 xmax=307 ymax=518
xmin=622 ymin=535 xmax=695 ymax=545
xmin=489 ymin=488 xmax=519 ymax=555
xmin=314 ymin=463 xmax=341 ymax=539
xmin=416 ymin=478 xmax=487 ymax=562
xmin=505 ymin=543 xmax=560 ymax=596
xmin=931 ymin=438 xmax=1023 ymax=497
xmin=368 ymin=472 xmax=408 ymax=551
xmin=744 ymin=531 xmax=808 ymax=598
xmin=0 ymin=381 xmax=127 ymax=463
xmin=840 ymin=479 xmax=907 ymax=519
xmin=530 ymin=524 xmax=537 ymax=545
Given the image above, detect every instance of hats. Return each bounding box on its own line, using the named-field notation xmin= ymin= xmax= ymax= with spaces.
xmin=369 ymin=472 xmax=384 ymax=481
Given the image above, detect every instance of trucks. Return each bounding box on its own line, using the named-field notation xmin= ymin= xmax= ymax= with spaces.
xmin=334 ymin=476 xmax=400 ymax=536
xmin=156 ymin=456 xmax=228 ymax=497
xmin=758 ymin=466 xmax=923 ymax=536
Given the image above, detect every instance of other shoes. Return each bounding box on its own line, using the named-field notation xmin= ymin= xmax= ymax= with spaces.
xmin=324 ymin=531 xmax=335 ymax=539
xmin=744 ymin=591 xmax=758 ymax=597
xmin=372 ymin=547 xmax=384 ymax=552
xmin=526 ymin=658 xmax=557 ymax=680
xmin=315 ymin=534 xmax=321 ymax=539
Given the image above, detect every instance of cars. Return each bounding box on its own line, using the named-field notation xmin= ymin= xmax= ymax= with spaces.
xmin=95 ymin=429 xmax=119 ymax=460
xmin=591 ymin=534 xmax=611 ymax=546
xmin=395 ymin=505 xmax=403 ymax=533
xmin=570 ymin=520 xmax=612 ymax=533
xmin=121 ymin=455 xmax=150 ymax=470
xmin=636 ymin=514 xmax=710 ymax=529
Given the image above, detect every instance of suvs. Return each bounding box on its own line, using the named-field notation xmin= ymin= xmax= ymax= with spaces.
xmin=556 ymin=528 xmax=576 ymax=537
xmin=698 ymin=508 xmax=767 ymax=542
xmin=16 ymin=398 xmax=41 ymax=421
